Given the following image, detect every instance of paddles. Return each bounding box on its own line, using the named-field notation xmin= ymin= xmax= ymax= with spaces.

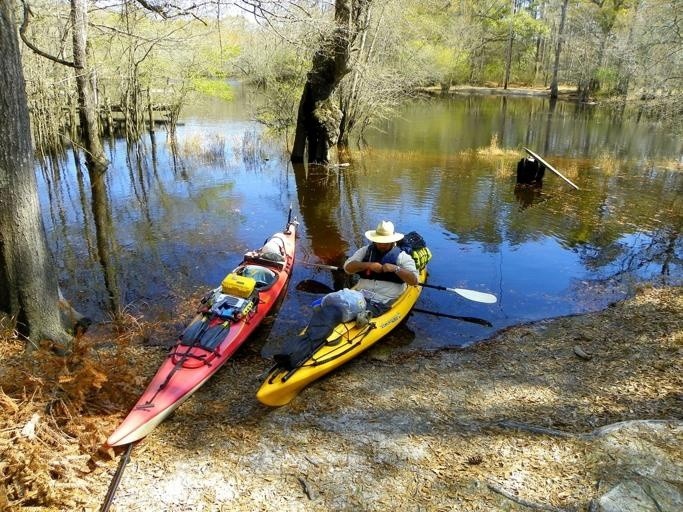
xmin=200 ymin=320 xmax=230 ymax=352
xmin=179 ymin=315 xmax=210 ymax=346
xmin=294 ymin=258 xmax=497 ymax=303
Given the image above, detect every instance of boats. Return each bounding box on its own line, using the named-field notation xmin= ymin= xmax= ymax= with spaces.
xmin=253 ymin=231 xmax=430 ymax=412
xmin=99 ymin=213 xmax=299 ymax=451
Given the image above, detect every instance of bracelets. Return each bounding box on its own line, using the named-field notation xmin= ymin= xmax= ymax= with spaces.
xmin=393 ymin=265 xmax=401 ymax=274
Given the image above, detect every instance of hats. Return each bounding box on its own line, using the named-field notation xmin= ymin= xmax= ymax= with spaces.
xmin=364 ymin=220 xmax=404 ymax=243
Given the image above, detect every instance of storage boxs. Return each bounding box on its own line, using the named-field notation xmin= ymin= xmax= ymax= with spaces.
xmin=221 ymin=273 xmax=256 ymax=298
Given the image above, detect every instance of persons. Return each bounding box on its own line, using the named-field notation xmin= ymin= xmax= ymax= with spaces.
xmin=342 ymin=220 xmax=419 ymax=301
